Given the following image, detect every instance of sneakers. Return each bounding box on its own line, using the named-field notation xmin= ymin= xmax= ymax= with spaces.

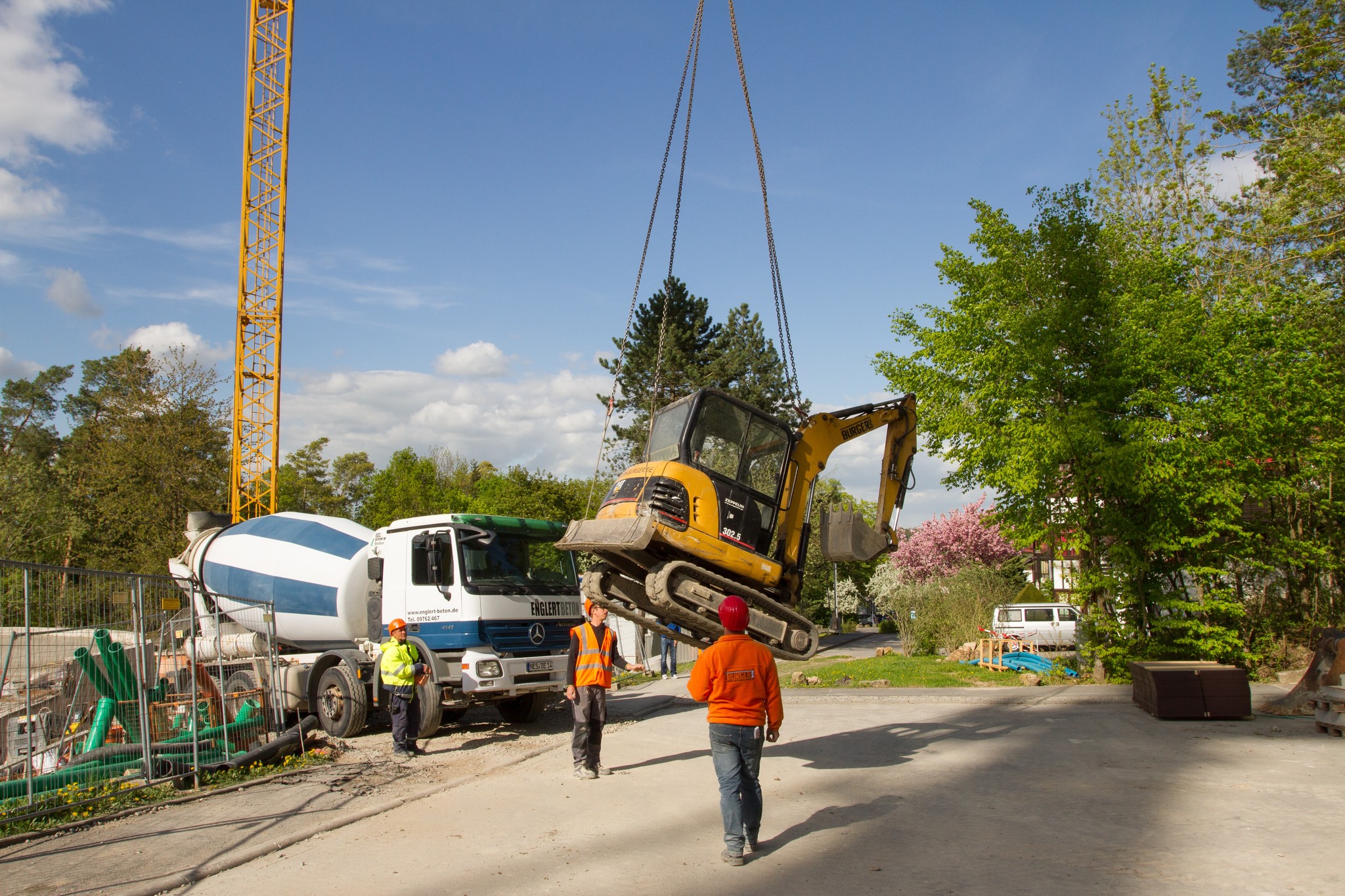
xmin=589 ymin=761 xmax=611 ymax=775
xmin=671 ymin=675 xmax=678 ymax=679
xmin=661 ymin=674 xmax=668 ymax=680
xmin=573 ymin=765 xmax=596 ymax=779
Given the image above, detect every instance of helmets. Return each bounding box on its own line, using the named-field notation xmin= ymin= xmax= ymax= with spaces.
xmin=584 ymin=598 xmax=596 ymax=616
xmin=387 ymin=619 xmax=407 ymax=636
xmin=718 ymin=595 xmax=750 ymax=630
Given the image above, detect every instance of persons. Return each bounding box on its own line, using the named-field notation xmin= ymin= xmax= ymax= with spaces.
xmin=380 ymin=618 xmax=432 ymax=758
xmin=657 ymin=621 xmax=681 ymax=680
xmin=686 ymin=595 xmax=784 ymax=866
xmin=566 ymin=598 xmax=645 ymax=779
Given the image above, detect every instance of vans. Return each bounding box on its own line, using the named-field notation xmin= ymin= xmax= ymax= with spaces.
xmin=991 ymin=603 xmax=1097 ymax=651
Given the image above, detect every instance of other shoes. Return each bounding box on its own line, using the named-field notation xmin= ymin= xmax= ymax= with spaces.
xmin=408 ymin=744 xmax=426 ymax=755
xmin=395 ymin=750 xmax=414 ymax=758
xmin=721 ymin=849 xmax=744 ymax=866
xmin=745 ymin=843 xmax=758 ymax=852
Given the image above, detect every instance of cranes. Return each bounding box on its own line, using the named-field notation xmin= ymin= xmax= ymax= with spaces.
xmin=228 ymin=0 xmax=296 ymax=527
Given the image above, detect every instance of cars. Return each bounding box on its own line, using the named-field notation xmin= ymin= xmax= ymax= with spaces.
xmin=858 ymin=613 xmax=890 ymax=627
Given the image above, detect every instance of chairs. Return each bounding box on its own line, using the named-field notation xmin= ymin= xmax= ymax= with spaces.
xmin=483 ymin=552 xmax=499 ymax=579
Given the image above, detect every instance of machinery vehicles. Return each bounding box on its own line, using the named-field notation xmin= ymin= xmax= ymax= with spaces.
xmin=169 ymin=511 xmax=586 ymax=739
xmin=553 ymin=390 xmax=919 ymax=663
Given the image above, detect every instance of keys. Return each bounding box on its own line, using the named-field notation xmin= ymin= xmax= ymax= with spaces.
xmin=585 ymin=686 xmax=590 ymax=694
